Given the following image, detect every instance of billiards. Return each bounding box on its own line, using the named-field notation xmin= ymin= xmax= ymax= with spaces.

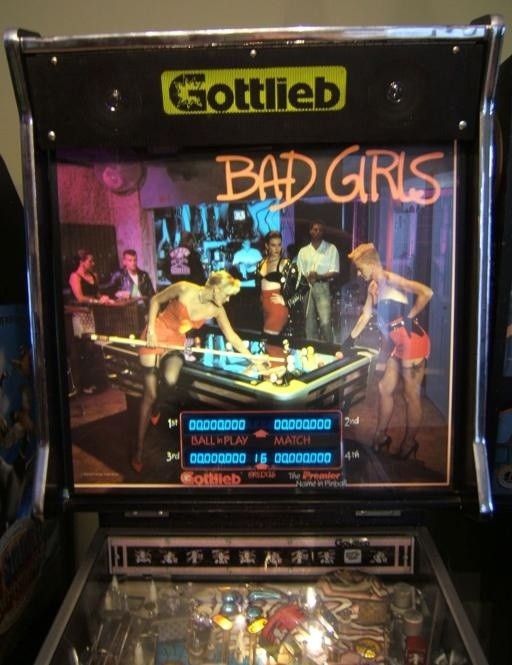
xmin=287 ymin=346 xmax=324 ymax=376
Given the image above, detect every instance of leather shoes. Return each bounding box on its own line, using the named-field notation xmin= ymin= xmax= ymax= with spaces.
xmin=132 ymin=461 xmax=143 ymax=473
xmin=150 ymin=412 xmax=161 ymax=427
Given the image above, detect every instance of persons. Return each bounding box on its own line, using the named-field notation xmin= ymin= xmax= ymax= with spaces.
xmin=340 ymin=241 xmax=434 ymax=458
xmin=163 ymin=232 xmax=209 ymax=286
xmin=234 ymin=238 xmax=263 ymax=280
xmin=288 ymin=242 xmax=298 ymax=261
xmin=67 ymin=249 xmax=115 ymax=306
xmin=254 ymin=231 xmax=312 ymax=336
xmin=129 ymin=268 xmax=270 ymax=472
xmin=297 ymin=217 xmax=340 ymax=342
xmin=110 ymin=249 xmax=156 ymax=303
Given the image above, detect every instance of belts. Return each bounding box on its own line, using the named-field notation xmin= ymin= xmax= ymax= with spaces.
xmin=316 ymin=278 xmax=328 ymax=281
xmin=387 ymin=315 xmax=418 ymax=331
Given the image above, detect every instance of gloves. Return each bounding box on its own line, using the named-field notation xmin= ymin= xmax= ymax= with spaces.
xmin=404 ymin=316 xmax=423 ymax=337
xmin=339 ymin=334 xmax=355 ymax=356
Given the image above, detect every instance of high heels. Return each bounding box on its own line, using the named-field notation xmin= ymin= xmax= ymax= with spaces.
xmin=390 ymin=441 xmax=419 ymax=461
xmin=364 ymin=435 xmax=392 ymax=455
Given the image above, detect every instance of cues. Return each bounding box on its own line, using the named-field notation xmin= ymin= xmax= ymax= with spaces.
xmin=91 ymin=335 xmax=283 ymax=361
